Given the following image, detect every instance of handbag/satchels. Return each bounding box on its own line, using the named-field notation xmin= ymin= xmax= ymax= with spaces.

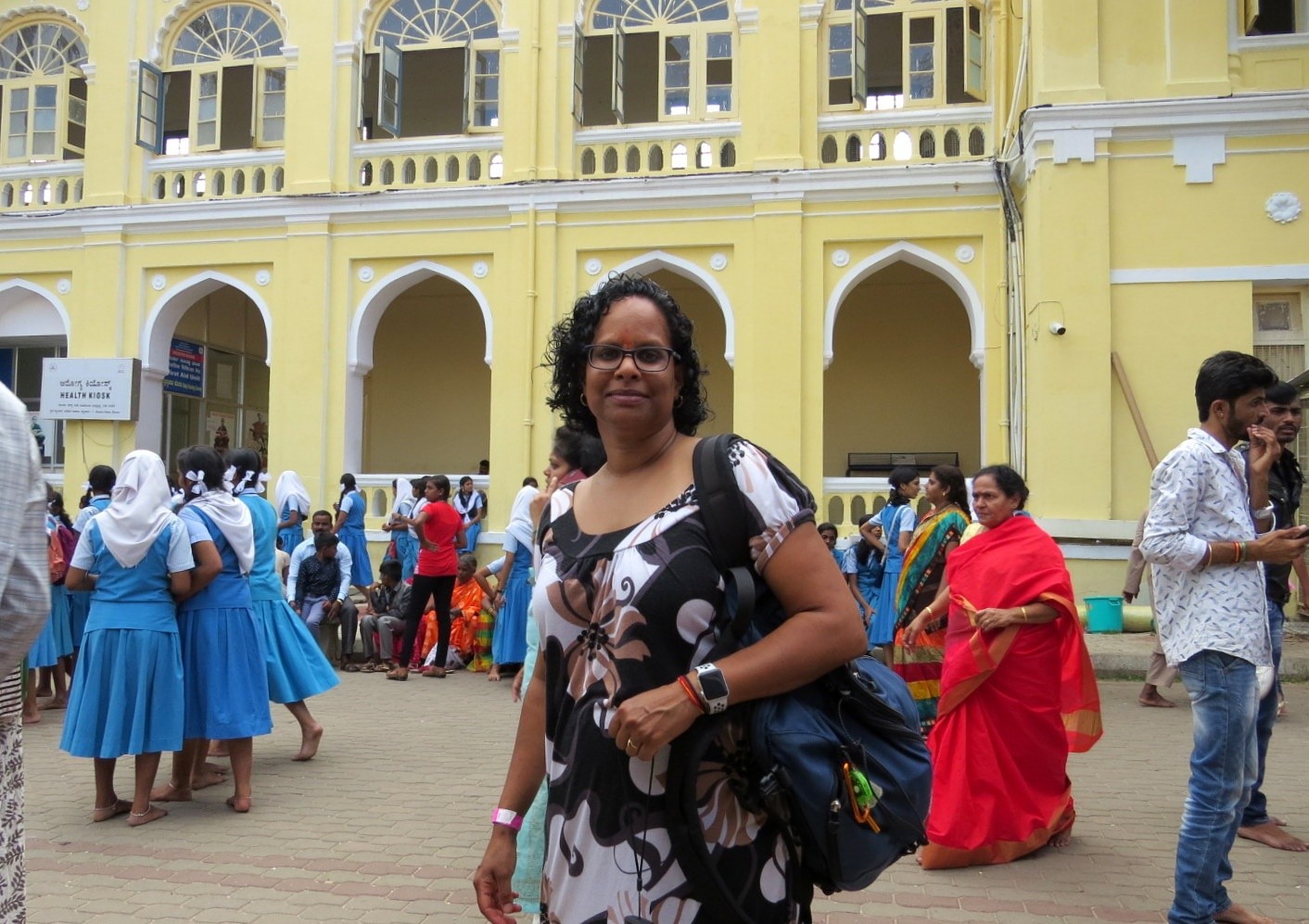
xmin=692 ymin=434 xmax=933 ymax=896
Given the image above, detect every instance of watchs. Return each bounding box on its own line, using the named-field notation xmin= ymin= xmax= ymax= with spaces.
xmin=1249 ymin=500 xmax=1274 ymax=521
xmin=496 ymin=589 xmax=504 ymax=594
xmin=696 ymin=662 xmax=730 ymax=715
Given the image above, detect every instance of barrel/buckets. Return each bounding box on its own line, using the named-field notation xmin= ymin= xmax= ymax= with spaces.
xmin=1083 ymin=598 xmax=1125 ymax=633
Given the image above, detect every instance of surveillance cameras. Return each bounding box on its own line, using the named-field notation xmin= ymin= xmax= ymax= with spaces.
xmin=1050 ymin=323 xmax=1066 ymax=335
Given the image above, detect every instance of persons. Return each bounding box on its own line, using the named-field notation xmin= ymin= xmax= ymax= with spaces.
xmin=816 ymin=464 xmax=1105 ymax=868
xmin=30 ymin=415 xmax=46 ymax=452
xmin=214 ymin=417 xmax=230 ymax=448
xmin=0 ymin=380 xmax=50 ymax=924
xmin=275 ymin=471 xmax=540 ymax=680
xmin=1122 ymin=350 xmax=1308 ymax=924
xmin=470 ymin=272 xmax=867 ymax=924
xmin=478 ymin=458 xmax=489 ymax=475
xmin=22 ymin=448 xmax=339 ymax=826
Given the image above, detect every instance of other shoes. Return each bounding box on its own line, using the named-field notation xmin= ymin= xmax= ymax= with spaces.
xmin=423 ymin=667 xmax=446 ymax=678
xmin=387 ymin=668 xmax=408 ymax=681
xmin=341 ymin=660 xmax=394 ymax=672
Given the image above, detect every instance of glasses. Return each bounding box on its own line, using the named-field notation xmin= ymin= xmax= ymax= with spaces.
xmin=583 ymin=345 xmax=681 ymax=372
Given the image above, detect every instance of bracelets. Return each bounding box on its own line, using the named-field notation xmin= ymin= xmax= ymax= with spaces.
xmin=678 ymin=675 xmax=707 ymax=714
xmin=1206 ymin=543 xmax=1213 ymax=568
xmin=488 ymin=607 xmax=492 ymax=612
xmin=1021 ymin=607 xmax=1029 ymax=624
xmin=1233 ymin=541 xmax=1242 ymax=563
xmin=490 ymin=609 xmax=494 ymax=614
xmin=491 ymin=808 xmax=524 ymax=830
xmin=399 ymin=514 xmax=402 ymax=520
xmin=1241 ymin=541 xmax=1246 ymax=561
xmin=926 ymin=607 xmax=935 ymax=623
xmin=336 ymin=599 xmax=343 ymax=605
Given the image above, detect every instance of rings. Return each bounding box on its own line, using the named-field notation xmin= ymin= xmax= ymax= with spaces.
xmin=628 ymin=739 xmax=638 ymax=750
